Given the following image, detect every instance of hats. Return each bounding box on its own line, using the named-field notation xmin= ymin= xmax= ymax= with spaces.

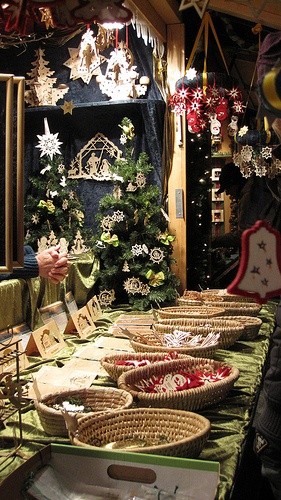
xmin=215 ymin=163 xmax=240 ymax=195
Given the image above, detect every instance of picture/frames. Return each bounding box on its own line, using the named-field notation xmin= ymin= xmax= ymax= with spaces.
xmin=212 ymin=210 xmax=224 ymax=223
xmin=212 ymin=189 xmax=224 ymax=201
xmin=212 ymin=168 xmax=222 ymax=181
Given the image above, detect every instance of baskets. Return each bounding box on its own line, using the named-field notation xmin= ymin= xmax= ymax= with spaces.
xmin=32 ymin=288 xmax=263 ymax=458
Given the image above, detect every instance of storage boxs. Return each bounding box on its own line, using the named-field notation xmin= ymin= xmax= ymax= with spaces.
xmin=0 ymin=289 xmax=262 ymax=500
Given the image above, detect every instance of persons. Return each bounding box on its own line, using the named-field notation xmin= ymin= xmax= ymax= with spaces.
xmin=0 ymin=246 xmax=70 ymax=284
